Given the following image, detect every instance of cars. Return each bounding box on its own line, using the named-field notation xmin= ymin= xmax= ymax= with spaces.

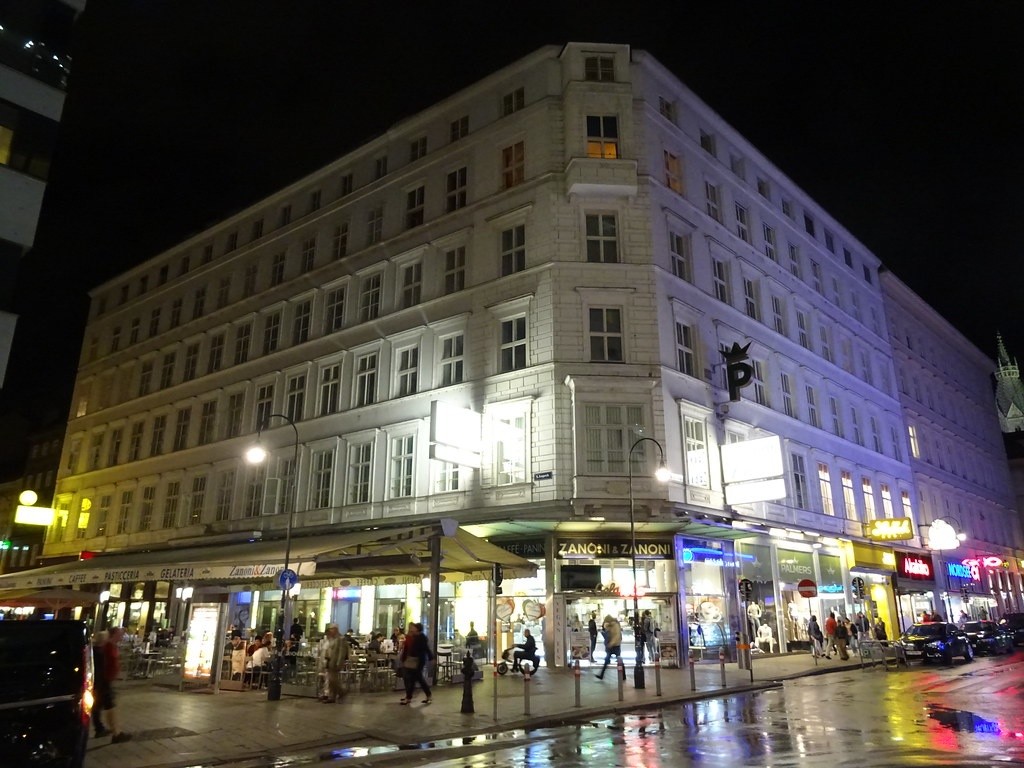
xmin=961 ymin=621 xmax=1014 ymax=656
xmin=0 ymin=619 xmax=95 ymax=768
xmin=894 ymin=622 xmax=974 ymax=667
xmin=1004 ymin=613 xmax=1024 ymax=644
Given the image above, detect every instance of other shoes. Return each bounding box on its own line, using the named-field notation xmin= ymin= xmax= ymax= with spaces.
xmin=318 ymin=695 xmax=336 ymax=704
xmin=595 ymin=675 xmax=602 ymax=679
xmin=591 ymin=658 xmax=597 ymax=662
xmin=401 ymin=698 xmax=411 ymax=702
xmin=112 ymin=731 xmax=133 ymax=744
xmin=510 ymin=667 xmax=519 ymax=673
xmin=93 ymin=728 xmax=112 ymax=738
xmin=622 ymin=676 xmax=626 ymax=680
xmin=421 ymin=699 xmax=431 ymax=704
xmin=812 ymin=650 xmax=837 ymax=659
xmin=400 ymin=699 xmax=410 ymax=705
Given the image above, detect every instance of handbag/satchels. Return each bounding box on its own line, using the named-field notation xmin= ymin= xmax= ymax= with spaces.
xmin=404 ymin=656 xmax=418 ymax=668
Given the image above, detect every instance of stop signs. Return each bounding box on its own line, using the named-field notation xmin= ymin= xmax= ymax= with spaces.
xmin=798 ymin=580 xmax=817 ymax=598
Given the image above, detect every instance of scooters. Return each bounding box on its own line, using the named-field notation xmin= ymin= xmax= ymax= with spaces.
xmin=497 ymin=646 xmax=540 ymax=676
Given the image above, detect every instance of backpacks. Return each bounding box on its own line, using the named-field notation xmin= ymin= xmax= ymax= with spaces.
xmin=645 ymin=617 xmax=655 ymax=632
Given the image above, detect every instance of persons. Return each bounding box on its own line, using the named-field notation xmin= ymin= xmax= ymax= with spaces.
xmin=688 ymin=599 xmax=730 ymax=646
xmin=923 ymin=611 xmax=944 ymax=624
xmin=94 ymin=618 xmax=436 ymax=704
xmin=589 ymin=613 xmax=598 ymax=662
xmin=638 ymin=609 xmax=662 ymax=663
xmin=978 ymin=606 xmax=989 ymax=621
xmin=808 ymin=611 xmax=889 ymax=660
xmin=510 ymin=629 xmax=536 ymax=673
xmin=594 ymin=615 xmax=626 ymax=680
xmin=89 ymin=627 xmax=130 ymax=743
xmin=958 ymin=610 xmax=968 ymax=628
xmin=278 ymin=609 xmax=317 ymax=635
xmin=466 ymin=621 xmax=480 ymax=645
xmin=747 ymin=601 xmax=843 ymax=654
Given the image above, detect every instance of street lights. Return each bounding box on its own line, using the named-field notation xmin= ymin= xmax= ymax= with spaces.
xmin=246 ymin=414 xmax=299 ymax=703
xmin=629 ymin=438 xmax=671 ymax=690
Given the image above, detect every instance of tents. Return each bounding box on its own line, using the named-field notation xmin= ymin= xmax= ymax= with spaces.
xmin=0 ymin=516 xmax=540 ymax=604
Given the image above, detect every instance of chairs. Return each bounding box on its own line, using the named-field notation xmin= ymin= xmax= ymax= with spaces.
xmin=116 ymin=634 xmax=466 ymax=692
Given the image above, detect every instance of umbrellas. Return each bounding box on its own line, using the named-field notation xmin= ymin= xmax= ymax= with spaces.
xmin=0 ymin=587 xmax=100 ymax=619
